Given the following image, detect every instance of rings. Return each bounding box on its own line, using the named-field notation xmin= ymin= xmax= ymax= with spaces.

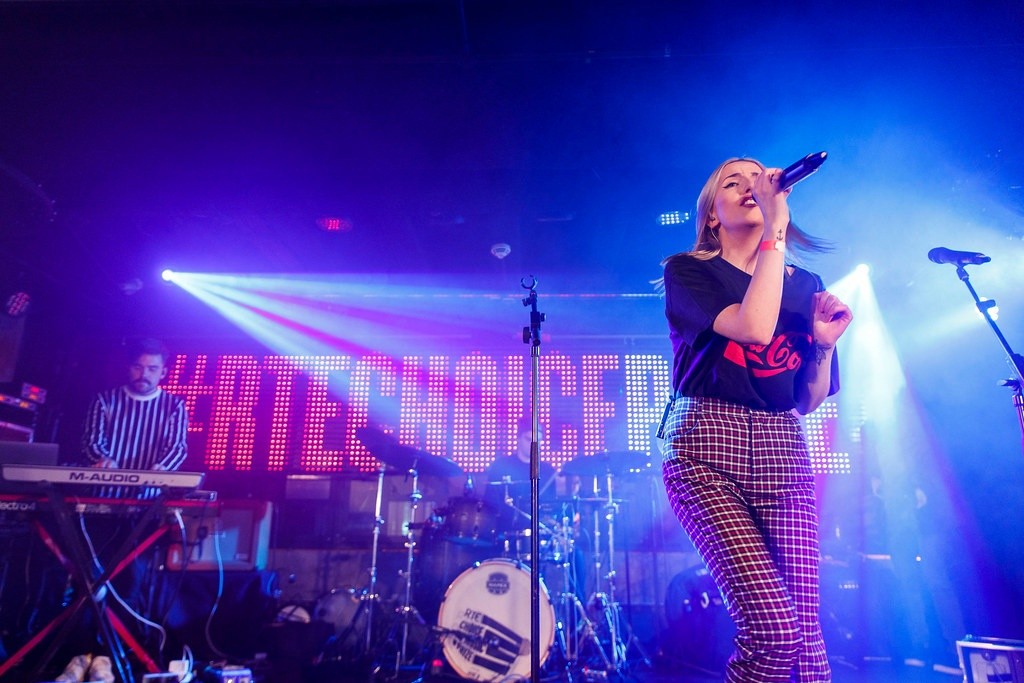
xmin=771 ymin=176 xmax=779 ymax=181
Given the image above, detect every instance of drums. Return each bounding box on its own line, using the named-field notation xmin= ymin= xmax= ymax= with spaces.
xmin=437 ymin=556 xmax=555 ymax=683
xmin=432 ymin=496 xmax=502 ymax=578
xmin=310 ymin=586 xmax=383 ymax=655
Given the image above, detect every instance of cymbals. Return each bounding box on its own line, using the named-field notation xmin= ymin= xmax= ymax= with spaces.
xmin=394 ymin=444 xmax=463 ymax=476
xmin=562 ymin=450 xmax=650 ymax=477
xmin=355 ymin=426 xmax=399 ymax=464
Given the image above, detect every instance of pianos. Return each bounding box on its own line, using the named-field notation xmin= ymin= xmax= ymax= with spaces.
xmin=2 ymin=463 xmax=207 ymax=488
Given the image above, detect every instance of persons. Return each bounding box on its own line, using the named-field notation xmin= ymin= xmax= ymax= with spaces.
xmin=54 ymin=342 xmax=190 ymax=683
xmin=481 ymin=419 xmax=591 ymax=670
xmin=656 ymin=158 xmax=853 ymax=683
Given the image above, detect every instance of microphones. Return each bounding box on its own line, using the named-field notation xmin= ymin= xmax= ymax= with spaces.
xmin=928 ymin=247 xmax=991 ymax=265
xmin=751 ymin=151 xmax=827 ymax=202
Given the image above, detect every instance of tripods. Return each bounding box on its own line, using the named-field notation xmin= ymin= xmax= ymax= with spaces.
xmin=312 ymin=456 xmax=657 ymax=683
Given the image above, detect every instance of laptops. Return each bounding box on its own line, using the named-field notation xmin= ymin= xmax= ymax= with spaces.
xmin=0 ymin=441 xmax=59 ymax=494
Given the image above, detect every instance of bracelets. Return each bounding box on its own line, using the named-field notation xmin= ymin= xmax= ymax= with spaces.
xmin=760 ymin=239 xmax=788 ymax=253
